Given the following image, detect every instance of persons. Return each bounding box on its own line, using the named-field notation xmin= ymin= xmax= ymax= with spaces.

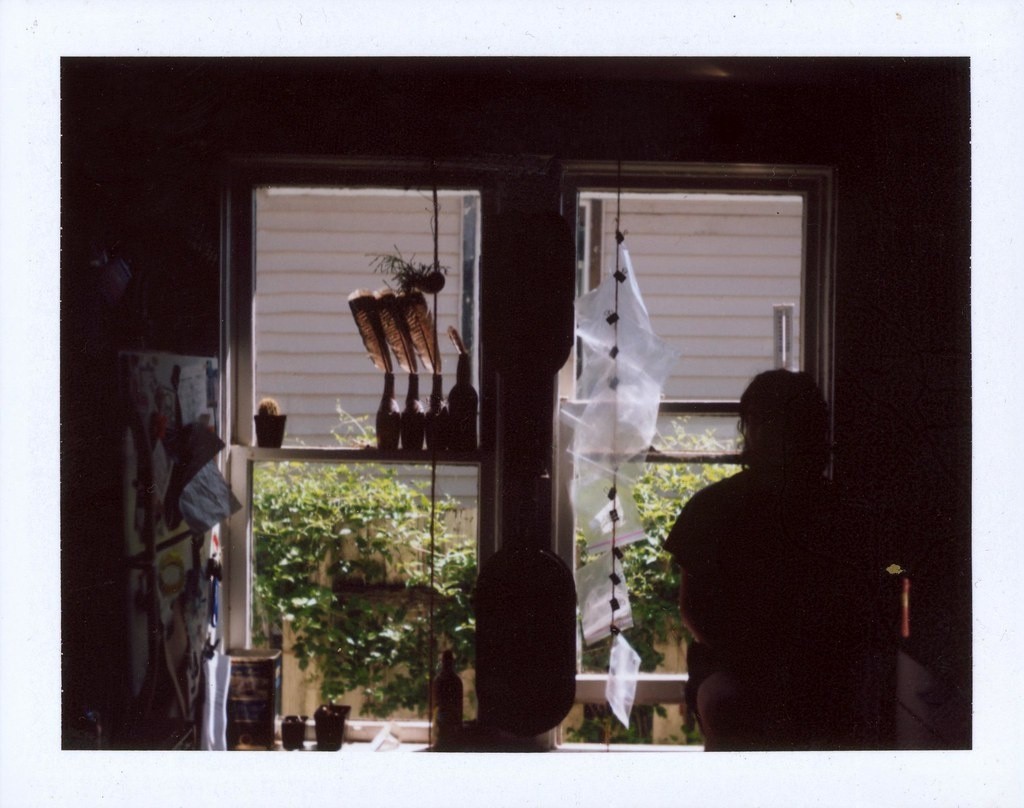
xmin=662 ymin=368 xmax=885 ymax=751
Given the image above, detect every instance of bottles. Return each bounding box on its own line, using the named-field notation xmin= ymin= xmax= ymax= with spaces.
xmin=448 ymin=353 xmax=477 ymax=454
xmin=426 ymin=373 xmax=448 ymax=452
xmin=401 ymin=372 xmax=425 ymax=451
xmin=376 ymin=373 xmax=401 ymax=452
xmin=431 ymin=649 xmax=466 ymax=752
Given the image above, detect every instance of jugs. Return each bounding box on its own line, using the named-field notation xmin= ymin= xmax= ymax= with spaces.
xmin=282 ymin=715 xmax=308 ymax=750
xmin=315 ymin=702 xmax=351 ymax=751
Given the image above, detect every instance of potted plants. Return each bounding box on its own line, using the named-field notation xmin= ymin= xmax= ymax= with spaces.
xmin=254 ymin=398 xmax=288 ymax=449
